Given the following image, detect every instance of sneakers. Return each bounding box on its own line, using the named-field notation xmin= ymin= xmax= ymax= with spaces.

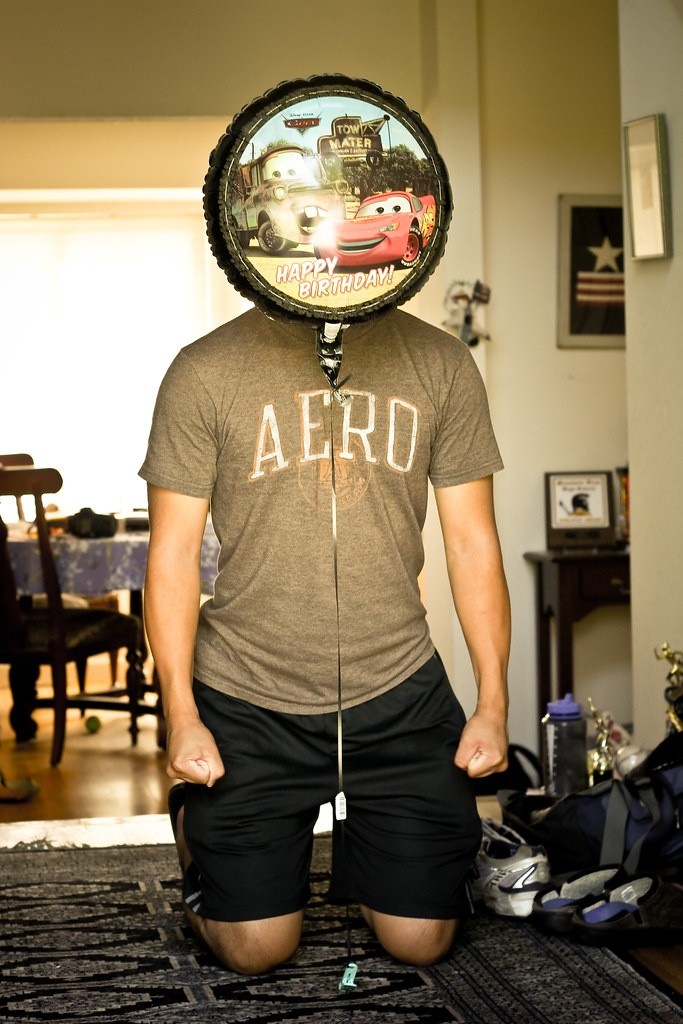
xmin=474 ymin=824 xmax=553 ymax=918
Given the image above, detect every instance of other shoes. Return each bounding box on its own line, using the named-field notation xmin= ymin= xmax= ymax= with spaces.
xmin=167 ymin=779 xmax=192 ymax=845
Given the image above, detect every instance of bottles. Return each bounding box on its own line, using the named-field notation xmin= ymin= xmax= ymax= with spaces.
xmin=546 ymin=693 xmax=589 ymax=797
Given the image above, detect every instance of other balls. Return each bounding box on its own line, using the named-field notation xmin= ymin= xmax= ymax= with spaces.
xmin=85 ymin=716 xmax=103 ymax=733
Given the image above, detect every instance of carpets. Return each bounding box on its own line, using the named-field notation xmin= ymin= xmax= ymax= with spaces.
xmin=0 ymin=835 xmax=682 ymax=1022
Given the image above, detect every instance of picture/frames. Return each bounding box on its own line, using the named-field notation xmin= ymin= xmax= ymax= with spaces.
xmin=551 ymin=188 xmax=630 ymax=350
xmin=618 ymin=110 xmax=673 ymax=263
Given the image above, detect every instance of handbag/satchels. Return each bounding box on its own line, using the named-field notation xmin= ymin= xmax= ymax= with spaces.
xmin=537 ymin=760 xmax=683 ymax=875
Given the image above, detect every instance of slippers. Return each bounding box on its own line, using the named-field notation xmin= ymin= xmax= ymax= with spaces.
xmin=569 ymin=872 xmax=683 ymax=947
xmin=531 ymin=862 xmax=631 ymax=933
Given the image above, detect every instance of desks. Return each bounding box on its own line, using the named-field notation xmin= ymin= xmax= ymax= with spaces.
xmin=6 ymin=510 xmax=219 ymax=748
xmin=521 ymin=545 xmax=634 ymax=782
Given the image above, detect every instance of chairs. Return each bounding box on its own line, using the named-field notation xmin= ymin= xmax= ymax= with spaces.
xmin=0 ymin=453 xmax=123 ymax=719
xmin=0 ymin=467 xmax=150 ymax=764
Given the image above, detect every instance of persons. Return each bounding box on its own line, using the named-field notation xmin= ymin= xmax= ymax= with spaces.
xmin=137 ymin=307 xmax=510 ymax=974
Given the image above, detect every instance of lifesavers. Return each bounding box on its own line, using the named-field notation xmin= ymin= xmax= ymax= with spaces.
xmin=321 ymin=151 xmax=339 ymax=172
xmin=366 ymin=149 xmax=383 ymax=169
